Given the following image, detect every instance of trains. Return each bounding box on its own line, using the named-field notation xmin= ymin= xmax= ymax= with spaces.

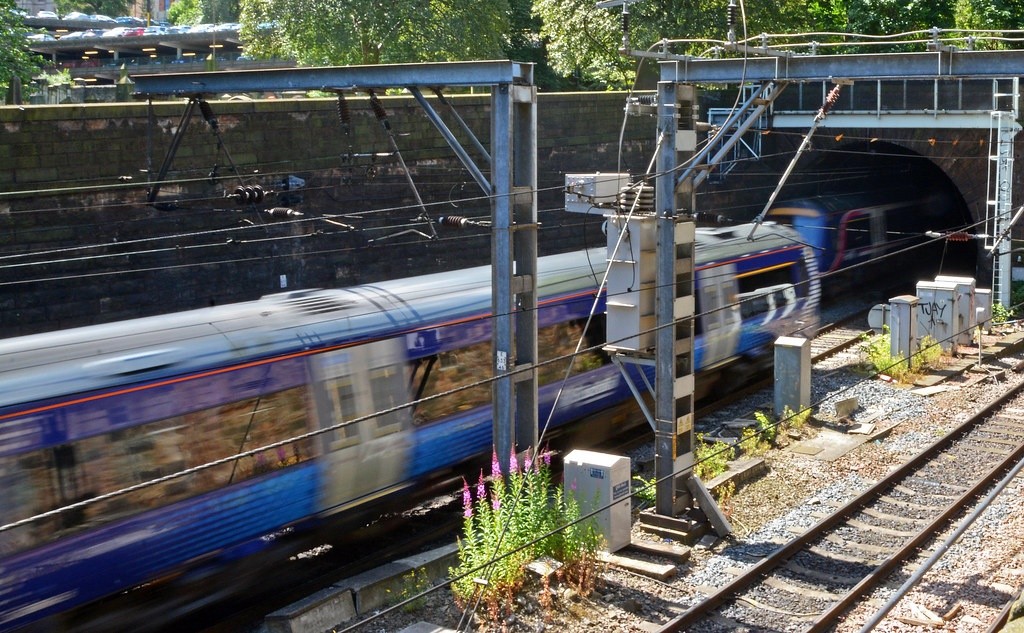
xmin=1 ymin=221 xmax=821 ymax=633
xmin=763 ymin=191 xmax=961 ymax=311
